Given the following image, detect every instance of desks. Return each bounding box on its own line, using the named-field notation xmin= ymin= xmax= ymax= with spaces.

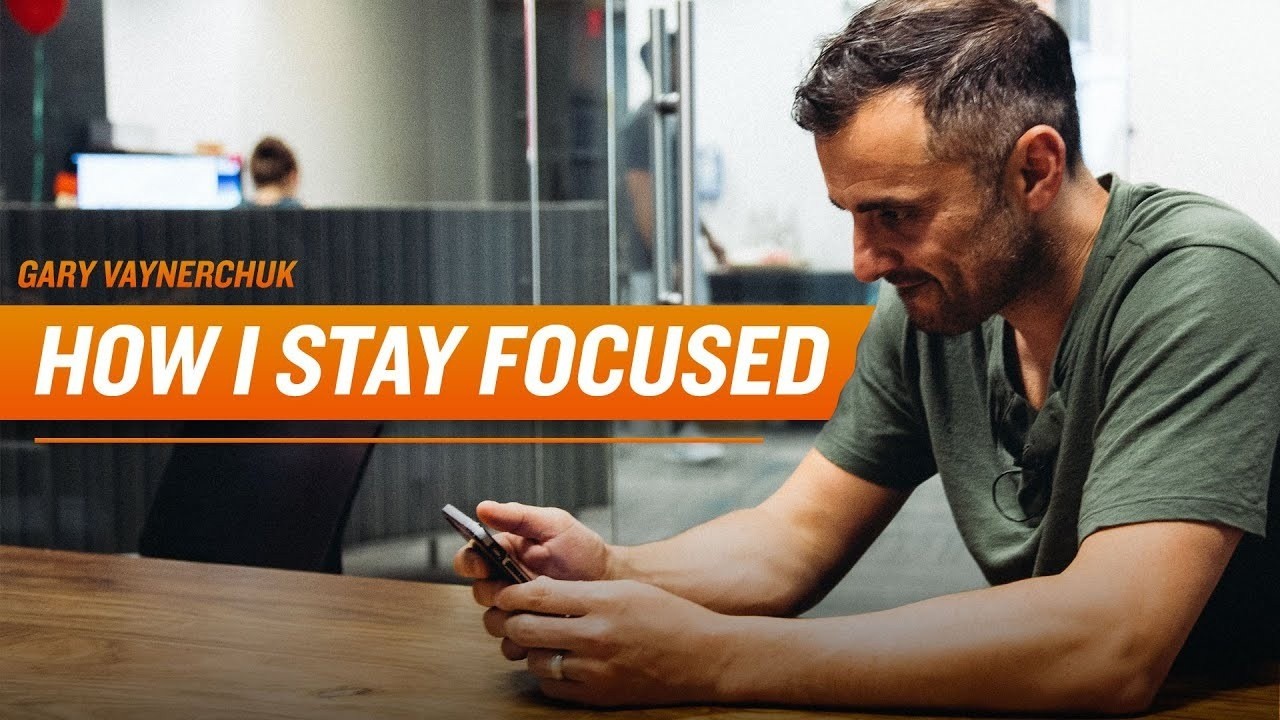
xmin=0 ymin=546 xmax=1279 ymax=720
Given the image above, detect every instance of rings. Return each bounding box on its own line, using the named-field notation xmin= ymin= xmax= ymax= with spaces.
xmin=553 ymin=651 xmax=565 ymax=681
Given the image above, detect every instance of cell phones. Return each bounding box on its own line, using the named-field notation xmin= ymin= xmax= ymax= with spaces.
xmin=442 ymin=504 xmax=531 ymax=586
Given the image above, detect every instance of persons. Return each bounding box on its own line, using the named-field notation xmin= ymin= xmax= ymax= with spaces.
xmin=618 ymin=36 xmax=735 ymax=464
xmin=455 ymin=0 xmax=1280 ymax=714
xmin=230 ymin=137 xmax=306 ymax=208
